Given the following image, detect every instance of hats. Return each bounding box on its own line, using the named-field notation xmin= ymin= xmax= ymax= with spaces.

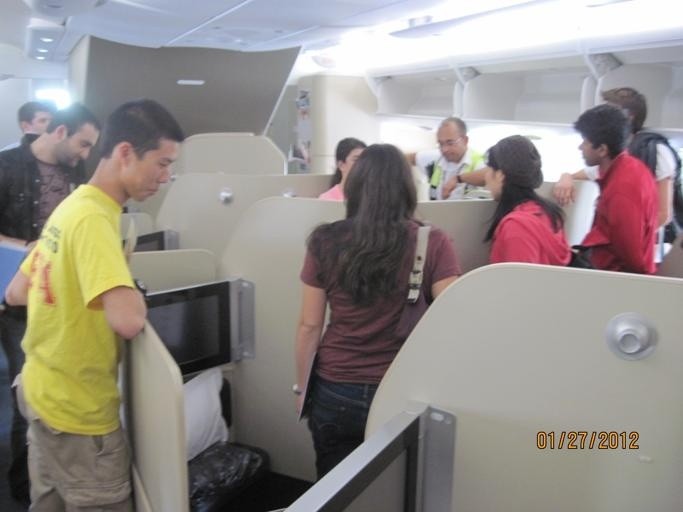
xmin=488 ymin=136 xmax=542 ymax=190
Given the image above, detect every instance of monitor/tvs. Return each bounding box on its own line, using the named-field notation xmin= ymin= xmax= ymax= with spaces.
xmin=123 ymin=230 xmax=167 ymax=251
xmin=277 ymin=402 xmax=426 ymax=512
xmin=144 ymin=277 xmax=240 ymax=383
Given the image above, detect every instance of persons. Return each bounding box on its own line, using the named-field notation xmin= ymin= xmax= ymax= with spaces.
xmin=552 ymin=88 xmax=683 ymax=269
xmin=294 ymin=145 xmax=462 ymax=487
xmin=405 ymin=117 xmax=489 ymax=201
xmin=0 ymin=99 xmax=58 ymax=152
xmin=0 ymin=103 xmax=103 ymax=251
xmin=318 ymin=138 xmax=368 ymax=201
xmin=567 ymin=104 xmax=660 ymax=276
xmin=483 ymin=135 xmax=571 ymax=266
xmin=5 ymin=98 xmax=185 ymax=512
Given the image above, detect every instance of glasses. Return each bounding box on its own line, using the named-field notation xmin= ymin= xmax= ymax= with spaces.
xmin=437 ymin=136 xmax=465 ymax=147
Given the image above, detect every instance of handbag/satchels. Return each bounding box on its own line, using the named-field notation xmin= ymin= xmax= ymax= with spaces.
xmin=395 ymin=225 xmax=432 ymax=340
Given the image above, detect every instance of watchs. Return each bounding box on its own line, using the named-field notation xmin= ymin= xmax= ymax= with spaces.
xmin=457 ymin=174 xmax=462 ymax=183
xmin=135 ymin=278 xmax=148 ymax=297
xmin=25 ymin=239 xmax=33 ymax=247
xmin=293 ymin=384 xmax=302 ymax=397
